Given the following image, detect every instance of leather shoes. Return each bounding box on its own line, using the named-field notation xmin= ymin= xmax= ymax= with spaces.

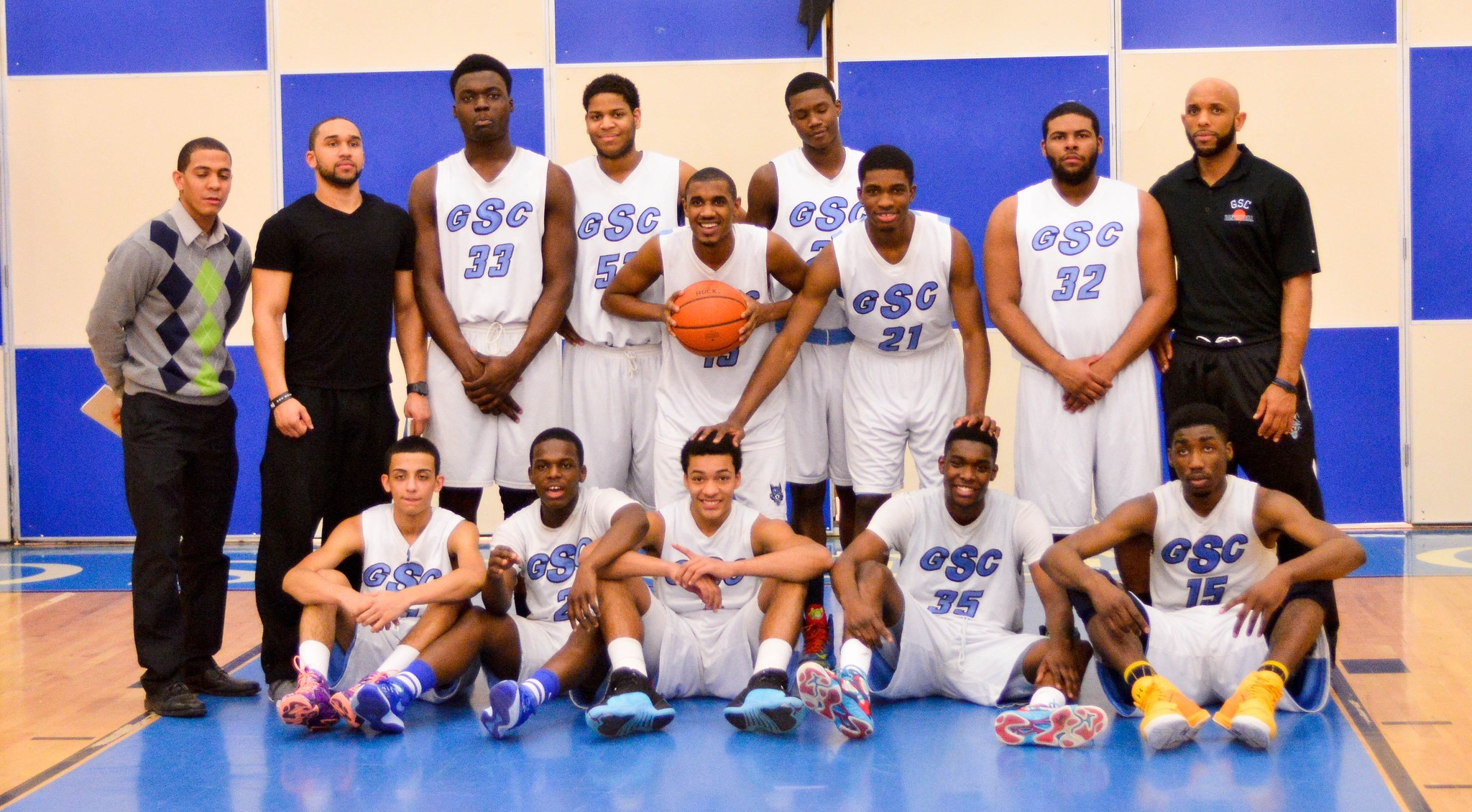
xmin=185 ymin=665 xmax=262 ymax=697
xmin=144 ymin=682 xmax=207 ymax=717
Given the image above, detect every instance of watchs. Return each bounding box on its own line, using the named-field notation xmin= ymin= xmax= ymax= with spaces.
xmin=406 ymin=380 xmax=429 ymax=396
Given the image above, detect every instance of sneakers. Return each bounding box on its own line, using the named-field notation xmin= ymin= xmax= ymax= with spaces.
xmin=797 ymin=602 xmax=836 ymax=669
xmin=584 ymin=668 xmax=676 ymax=738
xmin=724 ymin=668 xmax=809 ymax=734
xmin=994 ymin=698 xmax=1107 ymax=748
xmin=329 ymin=669 xmax=400 ymax=729
xmin=350 ymin=677 xmax=411 ymax=733
xmin=1212 ymin=670 xmax=1284 ymax=749
xmin=796 ymin=662 xmax=874 ymax=740
xmin=1131 ymin=675 xmax=1211 ymax=750
xmin=279 ymin=655 xmax=340 ymax=733
xmin=481 ymin=680 xmax=538 ymax=739
xmin=268 ymin=679 xmax=297 ymax=704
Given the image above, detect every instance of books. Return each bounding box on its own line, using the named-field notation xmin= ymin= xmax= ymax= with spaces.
xmin=80 ymin=384 xmax=123 ymax=439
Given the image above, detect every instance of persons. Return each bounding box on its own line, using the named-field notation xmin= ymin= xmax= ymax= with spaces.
xmin=982 ymin=102 xmax=1178 ymax=606
xmin=579 ymin=430 xmax=833 ymax=739
xmin=600 ymin=167 xmax=808 ymax=530
xmin=1148 ymin=77 xmax=1340 ymax=669
xmin=85 ymin=137 xmax=261 ymax=717
xmin=556 ymin=74 xmax=698 ymax=510
xmin=251 ymin=117 xmax=431 ymax=707
xmin=1039 ymin=403 xmax=1367 ymax=750
xmin=356 ymin=427 xmax=649 ymax=738
xmin=744 ymin=72 xmax=866 ymax=673
xmin=689 ymin=144 xmax=1000 ymax=566
xmin=276 ymin=435 xmax=487 ymax=731
xmin=409 ymin=54 xmax=576 ymax=617
xmin=796 ymin=422 xmax=1109 ymax=748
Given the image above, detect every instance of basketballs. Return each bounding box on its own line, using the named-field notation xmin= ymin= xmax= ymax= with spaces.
xmin=669 ymin=279 xmax=750 ymax=359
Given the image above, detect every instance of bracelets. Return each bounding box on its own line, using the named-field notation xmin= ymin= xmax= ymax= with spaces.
xmin=1271 ymin=376 xmax=1299 ymax=395
xmin=269 ymin=391 xmax=294 ymax=410
